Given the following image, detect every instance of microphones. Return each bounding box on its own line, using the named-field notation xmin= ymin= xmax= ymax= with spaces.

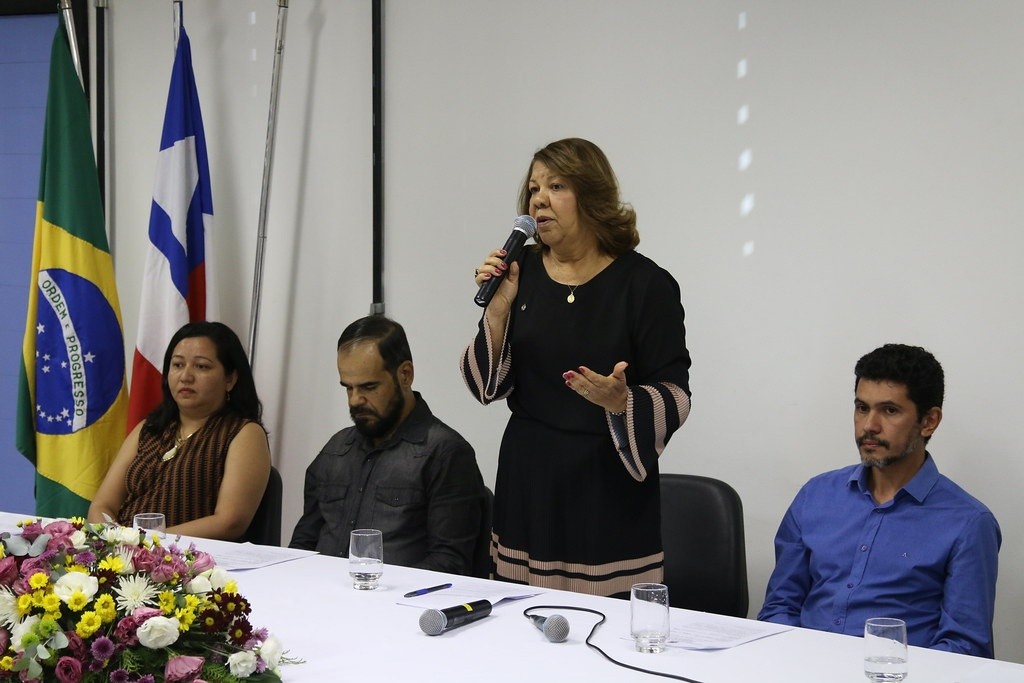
xmin=530 ymin=615 xmax=570 ymax=643
xmin=419 ymin=600 xmax=492 ymax=635
xmin=474 ymin=214 xmax=537 ymax=307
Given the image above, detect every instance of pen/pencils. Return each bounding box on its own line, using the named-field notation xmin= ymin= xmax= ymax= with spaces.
xmin=404 ymin=583 xmax=453 ymax=598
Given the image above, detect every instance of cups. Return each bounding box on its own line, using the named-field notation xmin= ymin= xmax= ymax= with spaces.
xmin=632 ymin=583 xmax=671 ymax=654
xmin=133 ymin=512 xmax=167 ymax=540
xmin=863 ymin=618 xmax=909 ymax=683
xmin=349 ymin=529 xmax=384 ymax=591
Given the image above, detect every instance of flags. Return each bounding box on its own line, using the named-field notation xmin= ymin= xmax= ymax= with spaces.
xmin=127 ymin=23 xmax=214 ymax=438
xmin=17 ymin=15 xmax=128 ymax=523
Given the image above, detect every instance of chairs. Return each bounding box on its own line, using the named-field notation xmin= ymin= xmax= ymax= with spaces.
xmin=659 ymin=473 xmax=751 ymax=618
xmin=241 ymin=464 xmax=283 ymax=546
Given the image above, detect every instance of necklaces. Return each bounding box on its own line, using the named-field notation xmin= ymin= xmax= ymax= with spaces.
xmin=161 ymin=431 xmax=193 ymax=462
xmin=558 ymin=267 xmax=587 ymax=303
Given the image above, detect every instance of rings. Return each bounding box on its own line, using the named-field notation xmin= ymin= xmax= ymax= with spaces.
xmin=583 ymin=390 xmax=589 ymax=396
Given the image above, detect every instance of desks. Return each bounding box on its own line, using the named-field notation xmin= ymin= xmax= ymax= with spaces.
xmin=0 ymin=511 xmax=1024 ymax=683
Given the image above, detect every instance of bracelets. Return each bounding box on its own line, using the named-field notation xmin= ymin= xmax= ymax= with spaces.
xmin=609 ymin=409 xmax=626 ymax=416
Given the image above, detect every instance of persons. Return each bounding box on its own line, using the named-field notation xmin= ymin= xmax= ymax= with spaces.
xmin=86 ymin=321 xmax=272 ymax=544
xmin=757 ymin=342 xmax=1002 ymax=658
xmin=288 ymin=315 xmax=489 ymax=579
xmin=460 ymin=138 xmax=692 ymax=600
